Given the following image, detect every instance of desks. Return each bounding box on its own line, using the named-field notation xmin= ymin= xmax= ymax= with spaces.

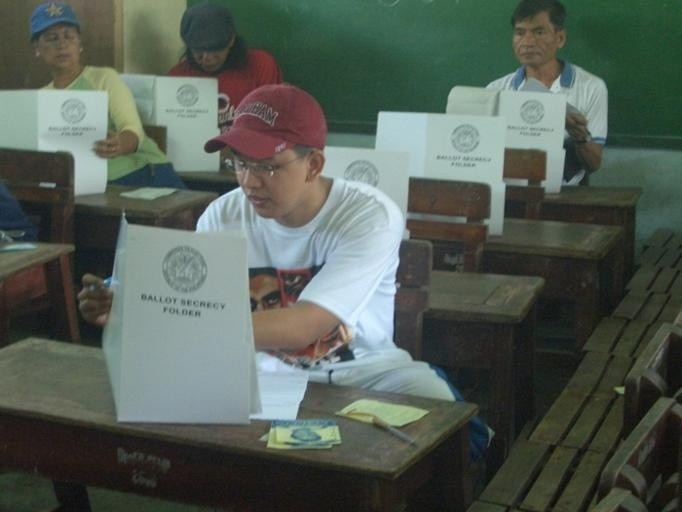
xmin=0 ymin=171 xmax=644 ymax=510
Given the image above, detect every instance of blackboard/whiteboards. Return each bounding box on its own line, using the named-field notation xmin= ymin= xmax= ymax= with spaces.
xmin=184 ymin=1 xmax=682 ymax=150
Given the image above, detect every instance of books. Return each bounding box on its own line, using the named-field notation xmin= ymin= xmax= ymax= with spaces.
xmin=117 ymin=74 xmax=221 ymax=174
xmin=0 ymin=88 xmax=110 ymax=198
xmin=101 ymin=208 xmax=264 ymax=425
xmin=446 ymin=85 xmax=568 ymax=197
xmin=375 ymin=110 xmax=508 ymax=240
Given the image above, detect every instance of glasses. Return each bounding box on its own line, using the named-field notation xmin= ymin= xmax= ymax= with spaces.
xmin=223 ymin=156 xmax=300 ymax=181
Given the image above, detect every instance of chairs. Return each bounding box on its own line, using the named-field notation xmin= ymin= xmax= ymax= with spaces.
xmin=504 ymin=148 xmax=548 ymax=220
xmin=143 ymin=125 xmax=166 ymax=153
xmin=1 ymin=147 xmax=77 ymax=350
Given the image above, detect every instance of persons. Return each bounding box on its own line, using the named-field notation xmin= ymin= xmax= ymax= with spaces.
xmin=0 ymin=182 xmax=40 ymax=244
xmin=167 ymin=2 xmax=286 ymax=161
xmin=249 ymin=266 xmax=281 ymax=312
xmin=29 ymin=3 xmax=192 ymax=191
xmin=280 ymin=272 xmax=343 ymax=352
xmin=485 ymin=2 xmax=609 ymax=186
xmin=78 ymin=82 xmax=506 ymax=489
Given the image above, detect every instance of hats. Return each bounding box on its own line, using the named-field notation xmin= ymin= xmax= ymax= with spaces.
xmin=180 ymin=2 xmax=237 ymax=52
xmin=28 ymin=3 xmax=81 ymax=39
xmin=202 ymin=82 xmax=328 ymax=161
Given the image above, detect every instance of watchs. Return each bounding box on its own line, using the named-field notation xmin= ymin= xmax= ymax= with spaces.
xmin=575 ymin=131 xmax=594 ymax=145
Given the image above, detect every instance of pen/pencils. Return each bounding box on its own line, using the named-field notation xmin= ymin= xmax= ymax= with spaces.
xmin=89 ymin=276 xmax=112 ymax=291
xmin=371 ymin=415 xmax=417 ymax=445
